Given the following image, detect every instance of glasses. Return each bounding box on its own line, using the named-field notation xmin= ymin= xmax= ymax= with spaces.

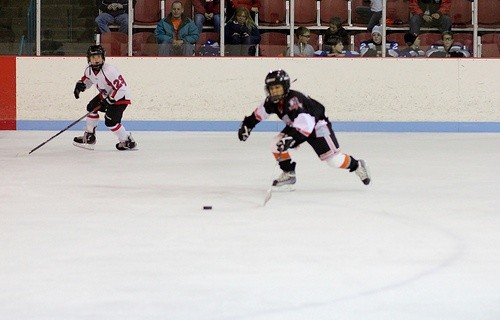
xmin=443 ymin=37 xmax=453 ymax=41
xmin=302 ymin=35 xmax=310 ymax=38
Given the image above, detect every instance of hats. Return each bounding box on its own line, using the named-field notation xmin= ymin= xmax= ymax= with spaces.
xmin=371 ymin=24 xmax=383 ymax=36
xmin=404 ymin=31 xmax=419 ymax=46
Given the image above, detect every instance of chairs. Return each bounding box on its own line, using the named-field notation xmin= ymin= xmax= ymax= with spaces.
xmin=99 ymin=0 xmax=500 ymax=57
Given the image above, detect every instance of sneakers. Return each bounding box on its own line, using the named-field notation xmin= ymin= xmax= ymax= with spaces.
xmin=347 ymin=155 xmax=371 ymax=186
xmin=272 ymin=161 xmax=297 ymax=192
xmin=116 ymin=132 xmax=137 ymax=151
xmin=73 ymin=126 xmax=96 ymax=150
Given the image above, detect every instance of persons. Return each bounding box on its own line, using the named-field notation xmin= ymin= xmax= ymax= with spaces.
xmin=427 ymin=31 xmax=471 ymax=57
xmin=322 ymin=15 xmax=350 ymax=52
xmin=153 ymin=1 xmax=200 ymax=56
xmin=313 ymin=36 xmax=358 ymax=56
xmin=404 ymin=0 xmax=452 ymax=44
xmin=222 ymin=6 xmax=261 ymax=45
xmin=227 ymin=0 xmax=264 ymax=23
xmin=356 ymin=0 xmax=383 ymax=33
xmin=285 ymin=26 xmax=314 ymax=56
xmin=359 ymin=26 xmax=399 ymax=56
xmin=94 ymin=0 xmax=136 ymax=34
xmin=238 ymin=69 xmax=371 ymax=185
xmin=192 ymin=0 xmax=225 ymax=32
xmin=398 ymin=32 xmax=425 ymax=57
xmin=74 ymin=45 xmax=137 ymax=151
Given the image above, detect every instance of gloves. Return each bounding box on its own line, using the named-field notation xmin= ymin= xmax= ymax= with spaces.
xmin=74 ymin=80 xmax=86 ymax=99
xmin=238 ymin=116 xmax=254 ymax=142
xmin=101 ymin=95 xmax=116 ymax=111
xmin=277 ymin=136 xmax=295 ymax=152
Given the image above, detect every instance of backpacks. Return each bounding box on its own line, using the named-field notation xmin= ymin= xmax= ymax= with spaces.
xmin=198 ymin=39 xmax=220 ymax=57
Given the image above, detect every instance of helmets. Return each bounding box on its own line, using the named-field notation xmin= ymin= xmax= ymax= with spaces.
xmin=265 ymin=70 xmax=290 ymax=103
xmin=87 ymin=45 xmax=105 ymax=70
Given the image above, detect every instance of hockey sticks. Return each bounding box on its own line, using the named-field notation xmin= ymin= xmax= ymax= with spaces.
xmin=28 ymin=105 xmax=102 ymax=155
xmin=262 ymin=147 xmax=282 ymax=205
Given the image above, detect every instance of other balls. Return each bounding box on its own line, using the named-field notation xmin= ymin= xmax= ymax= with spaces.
xmin=203 ymin=206 xmax=211 ymax=210
